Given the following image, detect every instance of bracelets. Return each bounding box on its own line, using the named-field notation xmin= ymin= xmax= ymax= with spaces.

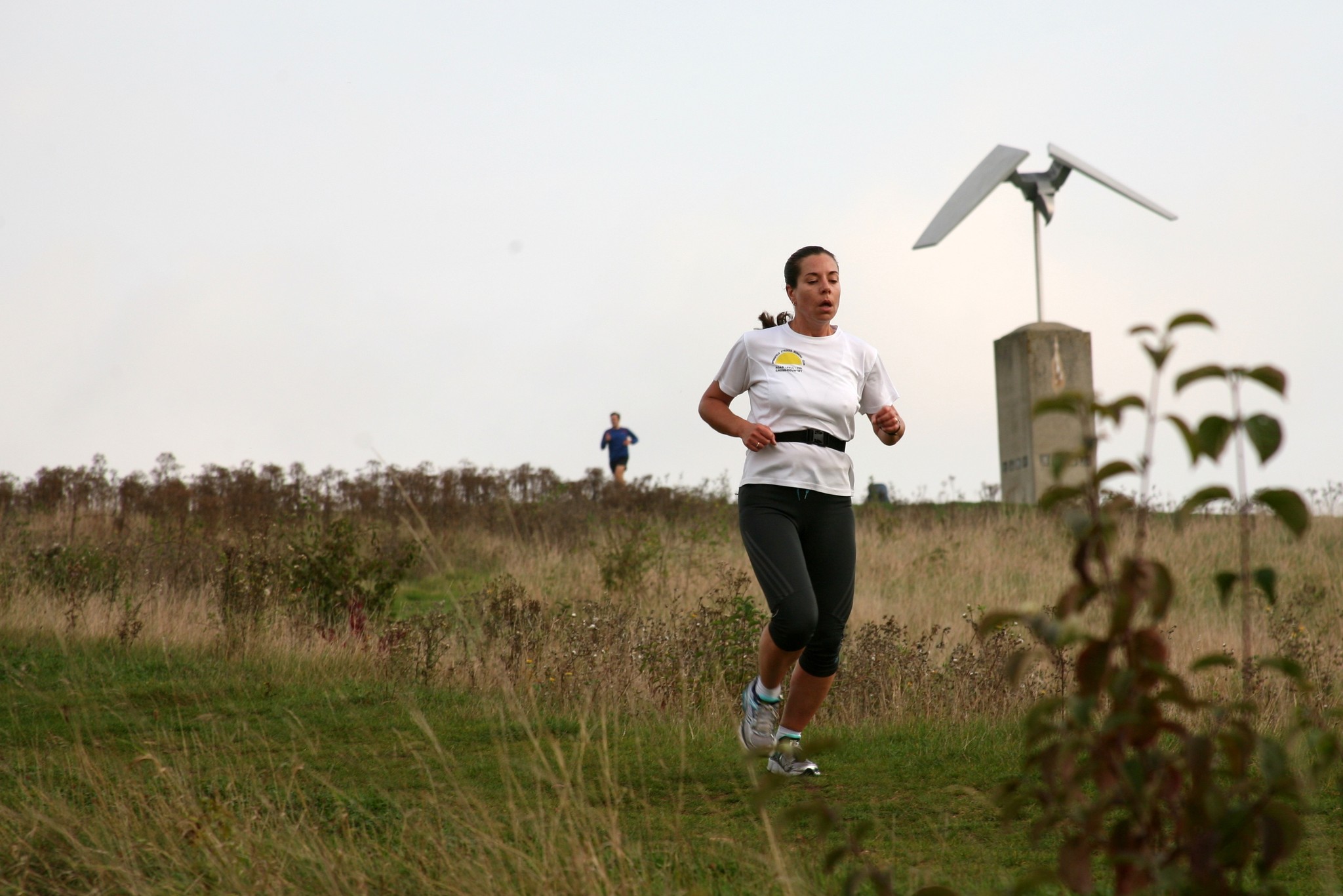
xmin=878 ymin=420 xmax=901 ymax=436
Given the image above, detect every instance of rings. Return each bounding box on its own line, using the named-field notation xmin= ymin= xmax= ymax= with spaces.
xmin=756 ymin=442 xmax=760 ymax=447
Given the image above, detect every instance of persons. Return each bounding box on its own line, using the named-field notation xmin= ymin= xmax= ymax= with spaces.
xmin=699 ymin=247 xmax=906 ymax=779
xmin=600 ymin=413 xmax=638 ymax=485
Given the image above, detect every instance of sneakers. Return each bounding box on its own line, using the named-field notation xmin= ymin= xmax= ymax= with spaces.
xmin=766 ymin=733 xmax=821 ymax=781
xmin=738 ymin=676 xmax=781 ymax=757
